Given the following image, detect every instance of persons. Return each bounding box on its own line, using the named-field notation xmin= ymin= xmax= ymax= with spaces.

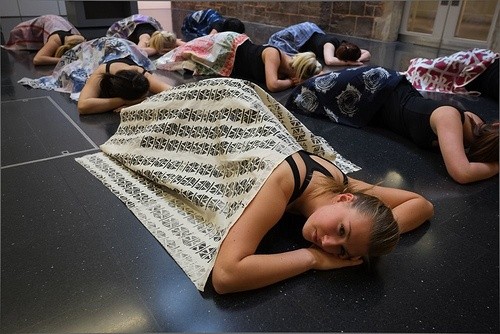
xmin=77 ymin=58 xmax=175 ymax=114
xmin=232 ymin=42 xmax=323 ymax=91
xmin=372 ymin=83 xmax=499 ymax=185
xmin=301 ymin=32 xmax=371 ymax=66
xmin=466 ymin=59 xmax=499 ymax=101
xmin=213 ymin=151 xmax=434 ymax=294
xmin=32 ymin=31 xmax=86 ymax=65
xmin=129 ymin=24 xmax=186 ymax=57
xmin=209 ymin=18 xmax=246 ymax=35
